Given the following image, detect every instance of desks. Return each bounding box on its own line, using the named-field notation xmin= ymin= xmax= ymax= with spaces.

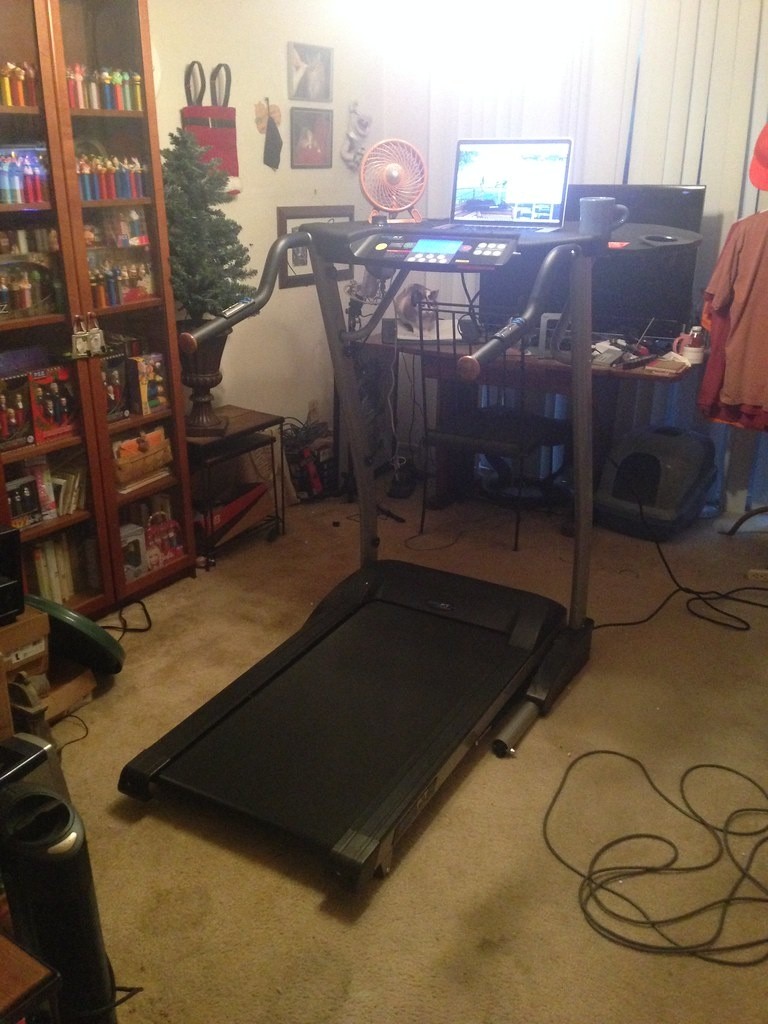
xmin=357 ymin=325 xmax=700 ymax=516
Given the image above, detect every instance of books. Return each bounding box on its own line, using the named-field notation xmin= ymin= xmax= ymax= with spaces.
xmin=5 ymin=449 xmax=88 ymax=607
xmin=644 ymin=356 xmax=687 ymax=374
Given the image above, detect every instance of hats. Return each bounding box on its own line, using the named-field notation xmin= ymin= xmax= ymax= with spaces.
xmin=748 ymin=122 xmax=768 ymax=191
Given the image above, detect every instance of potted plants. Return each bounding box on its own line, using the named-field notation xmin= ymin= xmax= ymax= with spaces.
xmin=158 ymin=124 xmax=262 ymax=436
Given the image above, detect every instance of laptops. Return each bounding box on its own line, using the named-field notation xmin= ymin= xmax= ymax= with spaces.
xmin=430 ymin=135 xmax=574 ymax=233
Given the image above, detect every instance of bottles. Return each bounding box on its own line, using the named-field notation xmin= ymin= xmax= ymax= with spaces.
xmin=682 ymin=325 xmax=705 ymax=366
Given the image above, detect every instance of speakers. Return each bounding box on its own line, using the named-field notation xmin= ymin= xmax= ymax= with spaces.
xmin=0 ymin=522 xmax=25 ymax=627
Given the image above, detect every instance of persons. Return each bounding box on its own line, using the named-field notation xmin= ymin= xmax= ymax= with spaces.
xmin=101 ymin=371 xmax=121 ymax=413
xmin=0 ymin=383 xmax=66 ymax=439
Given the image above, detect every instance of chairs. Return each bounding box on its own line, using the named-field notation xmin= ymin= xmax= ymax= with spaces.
xmin=414 ymin=291 xmax=565 ymax=554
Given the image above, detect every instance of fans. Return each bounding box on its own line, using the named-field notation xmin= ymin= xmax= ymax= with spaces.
xmin=358 ymin=137 xmax=431 ymax=225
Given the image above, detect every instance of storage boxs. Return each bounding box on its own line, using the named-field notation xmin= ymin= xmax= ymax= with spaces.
xmin=198 ymin=482 xmax=274 ymax=549
xmin=1 ymin=342 xmax=187 ymax=603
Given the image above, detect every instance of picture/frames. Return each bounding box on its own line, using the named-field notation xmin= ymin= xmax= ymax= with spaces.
xmin=275 ymin=37 xmax=357 ymax=288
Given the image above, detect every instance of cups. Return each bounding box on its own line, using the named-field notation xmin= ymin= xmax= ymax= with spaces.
xmin=578 ymin=196 xmax=630 ymax=249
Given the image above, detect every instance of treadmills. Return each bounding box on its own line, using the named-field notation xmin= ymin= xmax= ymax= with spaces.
xmin=115 ymin=199 xmax=638 ymax=899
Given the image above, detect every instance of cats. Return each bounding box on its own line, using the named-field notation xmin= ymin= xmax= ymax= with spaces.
xmin=395 ymin=283 xmax=445 ymax=337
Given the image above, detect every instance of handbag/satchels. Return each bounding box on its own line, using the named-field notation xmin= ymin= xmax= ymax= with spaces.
xmin=180 ymin=61 xmax=241 ymax=195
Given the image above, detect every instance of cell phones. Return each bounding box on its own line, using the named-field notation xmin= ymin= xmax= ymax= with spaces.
xmin=0 ymin=736 xmax=47 ymax=786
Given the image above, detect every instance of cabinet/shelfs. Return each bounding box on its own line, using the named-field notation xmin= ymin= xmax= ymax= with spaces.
xmin=183 ymin=405 xmax=286 ymax=568
xmin=0 ymin=3 xmax=199 ymax=623
